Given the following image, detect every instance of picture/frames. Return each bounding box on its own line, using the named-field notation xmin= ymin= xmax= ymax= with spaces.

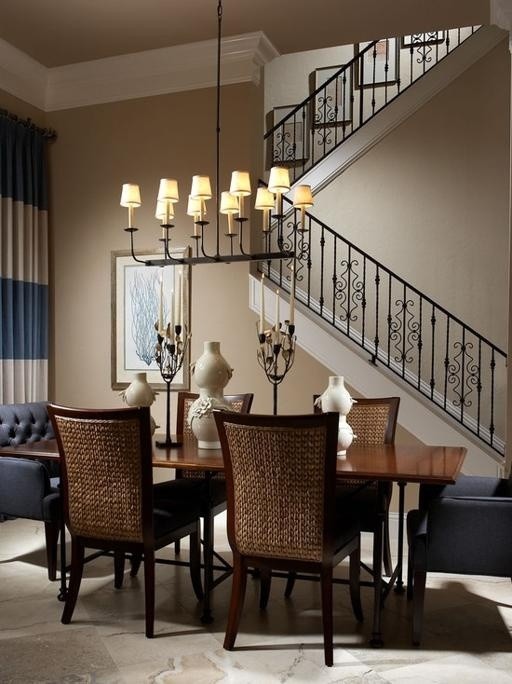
xmin=271 ymin=30 xmax=445 ymax=163
xmin=109 ymin=245 xmax=192 ymax=393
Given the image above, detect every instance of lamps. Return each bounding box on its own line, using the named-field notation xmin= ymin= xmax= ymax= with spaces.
xmin=119 ymin=1 xmax=315 ymax=265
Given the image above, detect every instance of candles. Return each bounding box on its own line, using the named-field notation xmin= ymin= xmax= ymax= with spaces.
xmin=259 ymin=269 xmax=294 ymax=333
xmin=158 ymin=274 xmax=182 ymax=343
